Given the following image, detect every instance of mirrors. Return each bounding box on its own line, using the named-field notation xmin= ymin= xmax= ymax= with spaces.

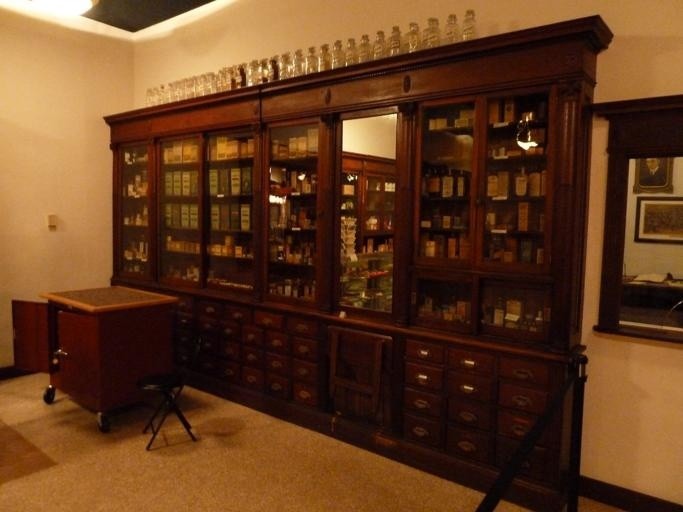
xmin=596 ymin=146 xmax=683 ymax=342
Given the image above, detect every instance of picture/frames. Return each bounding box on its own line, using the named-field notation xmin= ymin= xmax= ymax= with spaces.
xmin=633 ymin=158 xmax=673 ymax=194
xmin=634 ymin=196 xmax=683 ymax=244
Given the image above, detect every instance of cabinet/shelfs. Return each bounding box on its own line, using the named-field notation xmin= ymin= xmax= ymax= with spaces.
xmin=12 ymin=286 xmax=179 ymax=432
xmin=103 ymin=15 xmax=614 ymax=512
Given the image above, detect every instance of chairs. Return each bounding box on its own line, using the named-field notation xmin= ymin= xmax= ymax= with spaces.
xmin=143 ymin=311 xmax=199 ymax=449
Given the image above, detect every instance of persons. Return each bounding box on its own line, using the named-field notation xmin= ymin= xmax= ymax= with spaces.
xmin=641 ymin=158 xmax=665 ymax=184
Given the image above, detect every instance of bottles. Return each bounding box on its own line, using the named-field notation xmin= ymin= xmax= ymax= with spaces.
xmin=304 ymin=46 xmax=318 ymax=75
xmin=404 ymin=21 xmax=421 ymax=54
xmin=344 ymin=38 xmax=359 ymax=67
xmin=292 ymin=49 xmax=306 ymax=78
xmin=357 ymin=34 xmax=373 ymax=64
xmin=144 ymin=51 xmax=292 ymax=109
xmin=331 ymin=39 xmax=345 ymax=69
xmin=266 ymin=160 xmax=318 ymax=305
xmin=372 ymin=30 xmax=387 ymax=61
xmin=386 ymin=25 xmax=404 ymax=57
xmin=460 ymin=8 xmax=477 ymax=43
xmin=418 ymin=147 xmax=549 ymax=337
xmin=422 ymin=17 xmax=441 ymax=50
xmin=444 ymin=13 xmax=460 ymax=45
xmin=316 ymin=44 xmax=332 ymax=73
